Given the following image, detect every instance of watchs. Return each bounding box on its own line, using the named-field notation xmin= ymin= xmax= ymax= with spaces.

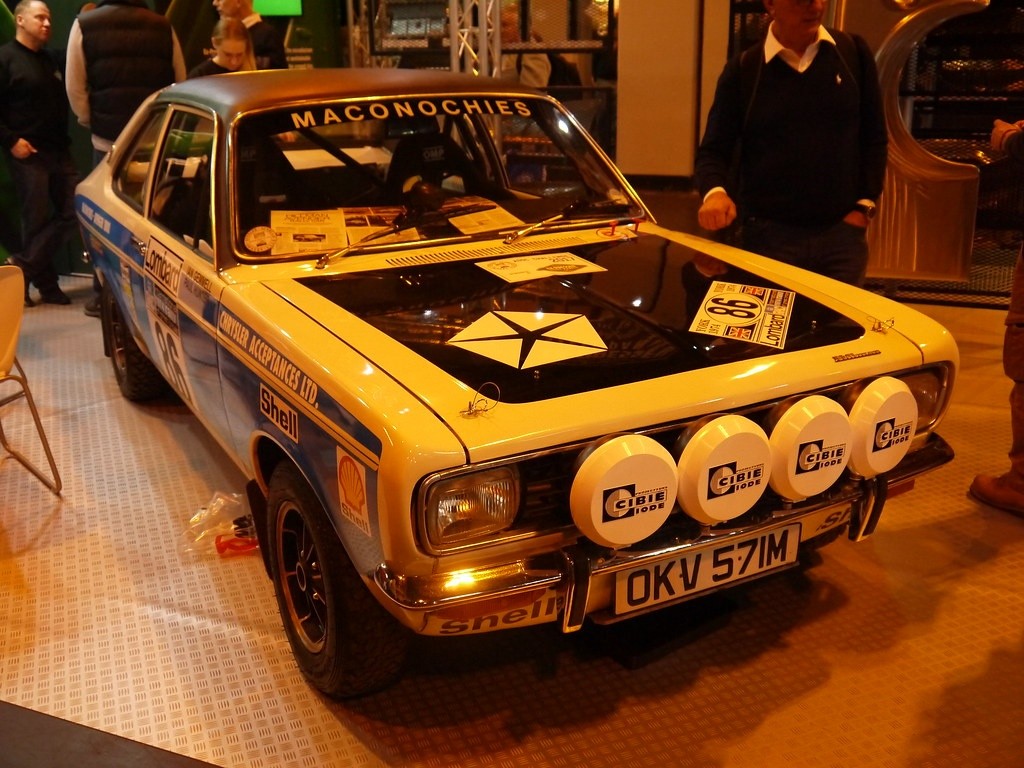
xmin=851 ymin=203 xmax=876 ymax=220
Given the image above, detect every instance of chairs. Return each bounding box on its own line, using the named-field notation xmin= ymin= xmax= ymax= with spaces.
xmin=181 ymin=132 xmax=318 ymax=231
xmin=0 ymin=264 xmax=62 ymax=495
xmin=383 ymin=133 xmax=479 ymax=201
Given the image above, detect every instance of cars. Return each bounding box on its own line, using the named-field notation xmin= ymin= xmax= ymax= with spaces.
xmin=500 ymin=85 xmax=615 ymax=198
xmin=73 ymin=66 xmax=973 ymax=705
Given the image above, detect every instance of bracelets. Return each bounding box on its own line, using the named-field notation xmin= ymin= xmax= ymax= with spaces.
xmin=1000 ymin=129 xmax=1020 ymax=152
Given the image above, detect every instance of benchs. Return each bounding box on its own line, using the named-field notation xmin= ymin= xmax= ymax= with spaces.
xmin=153 ymin=164 xmax=391 ymax=225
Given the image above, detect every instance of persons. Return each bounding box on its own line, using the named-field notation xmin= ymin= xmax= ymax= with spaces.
xmin=693 ymin=0 xmax=889 ymax=332
xmin=211 ymin=0 xmax=290 ymax=69
xmin=0 ymin=0 xmax=81 ymax=307
xmin=187 ymin=17 xmax=257 ymax=80
xmin=969 ymin=117 xmax=1024 ymax=515
xmin=478 ymin=0 xmax=585 ymax=101
xmin=63 ymin=0 xmax=187 ymax=317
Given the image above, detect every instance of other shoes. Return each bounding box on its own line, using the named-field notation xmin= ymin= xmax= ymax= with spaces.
xmin=39 ymin=282 xmax=71 ymax=305
xmin=969 ymin=473 xmax=1024 ymax=518
xmin=83 ymin=295 xmax=102 ymax=317
xmin=24 ymin=289 xmax=36 ymax=307
